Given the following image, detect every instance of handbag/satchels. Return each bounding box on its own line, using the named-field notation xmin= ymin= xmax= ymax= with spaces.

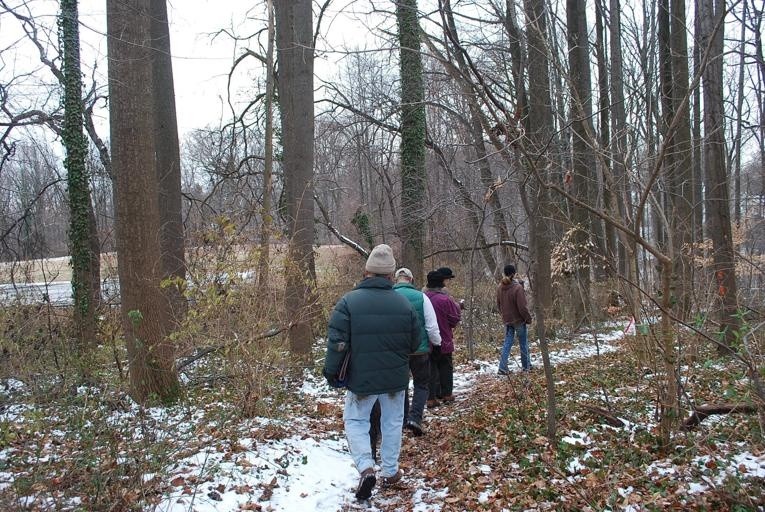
xmin=321 ymin=346 xmax=351 ymax=388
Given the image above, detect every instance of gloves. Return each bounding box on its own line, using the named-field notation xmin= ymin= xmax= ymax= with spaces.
xmin=429 ymin=346 xmax=442 ymax=362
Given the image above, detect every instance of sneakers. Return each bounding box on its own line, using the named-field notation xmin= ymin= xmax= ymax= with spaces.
xmin=427 ymin=398 xmax=441 ymax=408
xmin=379 ymin=469 xmax=405 ymax=488
xmin=356 ymin=467 xmax=377 ymax=500
xmin=405 ymin=420 xmax=426 ymax=437
xmin=442 ymin=394 xmax=456 ymax=406
xmin=498 ymin=368 xmax=513 ymax=376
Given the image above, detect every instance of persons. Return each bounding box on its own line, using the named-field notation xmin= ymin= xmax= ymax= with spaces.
xmin=495 ymin=264 xmax=536 ymax=375
xmin=323 ymin=242 xmax=422 ymax=502
xmin=361 ymin=266 xmax=464 ymax=471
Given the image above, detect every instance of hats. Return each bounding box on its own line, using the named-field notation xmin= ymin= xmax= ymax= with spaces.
xmin=395 ymin=268 xmax=414 ymax=284
xmin=365 ymin=243 xmax=397 ymax=274
xmin=426 ymin=267 xmax=455 ymax=288
